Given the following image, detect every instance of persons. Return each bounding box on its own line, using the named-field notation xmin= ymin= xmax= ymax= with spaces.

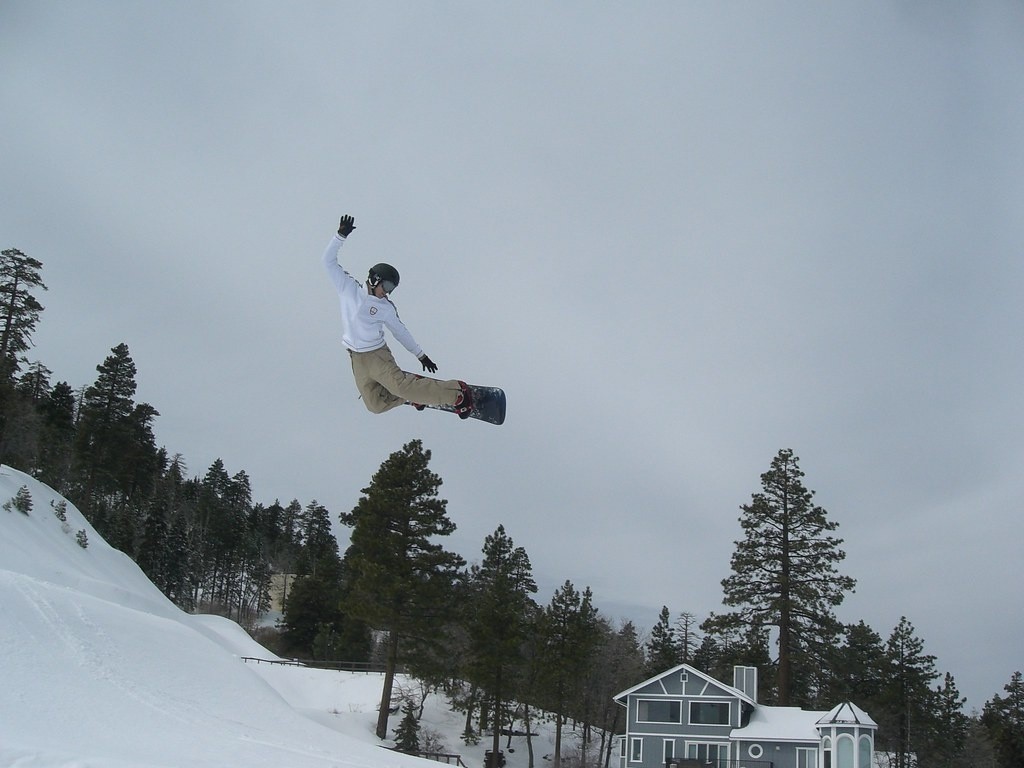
xmin=320 ymin=213 xmax=472 ymax=420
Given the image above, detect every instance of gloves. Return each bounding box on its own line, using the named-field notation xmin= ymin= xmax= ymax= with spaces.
xmin=418 ymin=354 xmax=438 ymax=373
xmin=337 ymin=214 xmax=356 ymax=239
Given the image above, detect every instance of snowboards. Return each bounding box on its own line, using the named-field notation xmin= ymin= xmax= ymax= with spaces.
xmin=402 ymin=370 xmax=506 ymax=426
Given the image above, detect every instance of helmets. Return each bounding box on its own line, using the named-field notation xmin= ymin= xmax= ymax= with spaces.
xmin=365 ymin=263 xmax=400 ymax=295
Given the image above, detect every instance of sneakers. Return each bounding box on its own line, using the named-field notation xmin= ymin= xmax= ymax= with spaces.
xmin=454 ymin=381 xmax=472 ymax=419
xmin=410 ymin=375 xmax=423 ymax=411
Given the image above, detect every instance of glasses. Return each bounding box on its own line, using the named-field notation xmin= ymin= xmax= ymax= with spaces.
xmin=381 ymin=279 xmax=395 ymax=295
